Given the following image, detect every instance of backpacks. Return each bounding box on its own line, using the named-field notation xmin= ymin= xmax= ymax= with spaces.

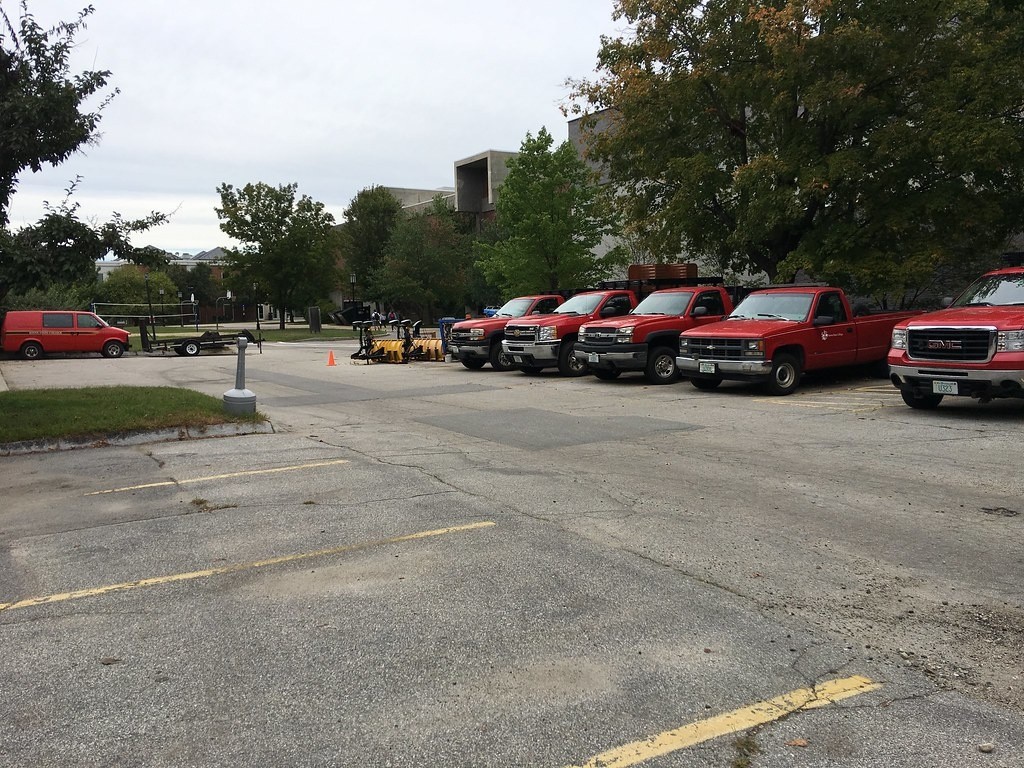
xmin=390 ymin=313 xmax=395 ymax=319
xmin=374 ymin=312 xmax=380 ymax=320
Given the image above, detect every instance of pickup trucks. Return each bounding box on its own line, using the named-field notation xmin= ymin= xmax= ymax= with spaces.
xmin=571 ymin=286 xmax=735 ymax=386
xmin=501 ymin=291 xmax=639 ymax=377
xmin=674 ymin=287 xmax=930 ymax=396
xmin=447 ymin=294 xmax=564 ymax=372
xmin=886 ymin=265 xmax=1024 ymax=408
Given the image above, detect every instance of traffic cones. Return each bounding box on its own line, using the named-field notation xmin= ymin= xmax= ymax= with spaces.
xmin=325 ymin=349 xmax=337 ymax=366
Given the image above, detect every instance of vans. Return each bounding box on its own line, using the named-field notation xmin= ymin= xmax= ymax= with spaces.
xmin=2 ymin=311 xmax=131 ymax=360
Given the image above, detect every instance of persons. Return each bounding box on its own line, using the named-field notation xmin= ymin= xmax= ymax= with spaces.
xmin=372 ymin=309 xmax=399 ymax=332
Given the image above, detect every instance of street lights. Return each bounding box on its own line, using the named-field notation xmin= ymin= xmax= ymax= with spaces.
xmin=350 ymin=273 xmax=356 ymax=321
xmin=159 ymin=289 xmax=165 ymax=327
xmin=178 ymin=291 xmax=183 ymax=327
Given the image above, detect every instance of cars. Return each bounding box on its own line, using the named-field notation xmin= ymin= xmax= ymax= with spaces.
xmin=485 ymin=306 xmax=501 ymax=315
